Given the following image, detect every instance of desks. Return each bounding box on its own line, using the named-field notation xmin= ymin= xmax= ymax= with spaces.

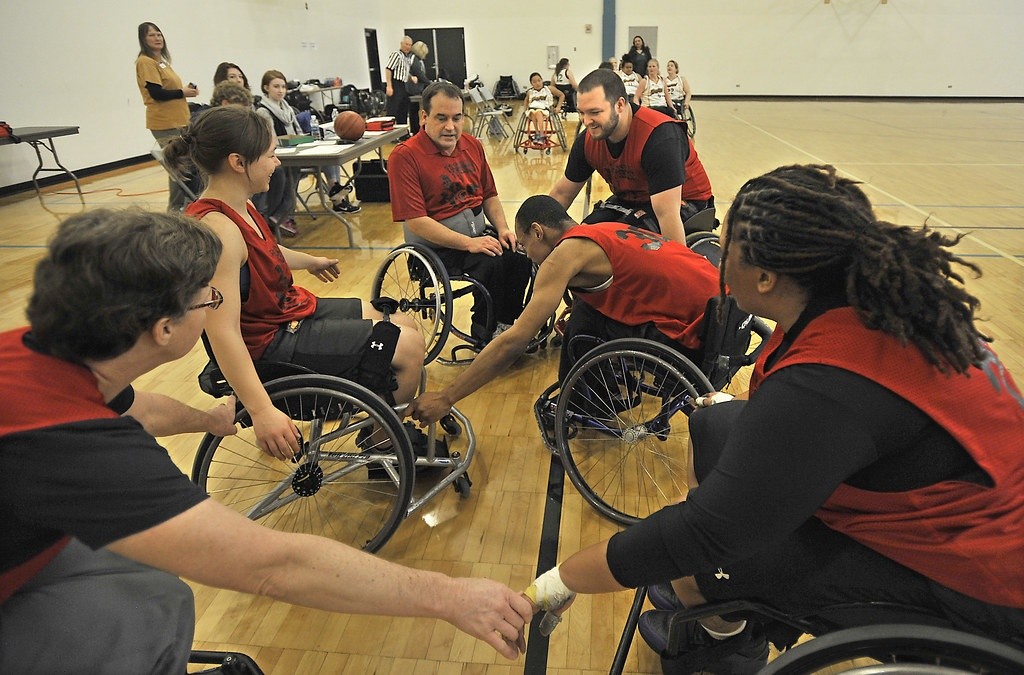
xmin=274 ymin=125 xmax=409 ymax=247
xmin=0 ymin=126 xmax=80 ymax=195
xmin=302 ymin=85 xmax=345 ymax=107
xmin=410 ymin=89 xmax=475 ymax=125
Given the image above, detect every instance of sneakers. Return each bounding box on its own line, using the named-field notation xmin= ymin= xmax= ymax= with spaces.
xmin=332 ymin=202 xmax=361 ymax=214
xmin=329 ymin=183 xmax=353 ymax=203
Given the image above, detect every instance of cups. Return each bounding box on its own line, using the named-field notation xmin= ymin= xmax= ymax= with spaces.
xmin=188 ymin=82 xmax=197 ymax=97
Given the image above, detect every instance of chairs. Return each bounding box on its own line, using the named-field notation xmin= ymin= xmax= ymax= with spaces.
xmin=469 ymin=87 xmax=515 ymax=142
xmin=151 ymin=150 xmax=326 ymax=236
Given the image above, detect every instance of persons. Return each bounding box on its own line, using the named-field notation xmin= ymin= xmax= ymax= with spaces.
xmin=211 ymin=81 xmax=251 ymax=109
xmin=524 ymin=72 xmax=564 ymax=144
xmin=598 ymin=54 xmax=642 ymax=103
xmin=548 ymin=69 xmax=713 ymax=237
xmin=501 ymin=164 xmax=1024 ymax=675
xmin=551 ymin=58 xmax=578 ymax=90
xmin=406 ymin=195 xmax=729 ymax=430
xmin=256 ymin=70 xmax=362 ymax=213
xmin=388 ymin=81 xmax=539 ymax=354
xmin=665 ymin=60 xmax=691 ymax=109
xmin=385 ymin=37 xmax=412 ymax=143
xmin=407 ymin=41 xmax=433 ymax=135
xmin=135 ymin=22 xmax=197 ymax=209
xmin=0 ymin=207 xmax=529 ymax=674
xmin=629 ymin=36 xmax=652 ymax=78
xmin=634 ymin=59 xmax=676 ymax=114
xmin=165 ymin=108 xmax=448 ymax=474
xmin=214 ymin=62 xmax=295 ymax=239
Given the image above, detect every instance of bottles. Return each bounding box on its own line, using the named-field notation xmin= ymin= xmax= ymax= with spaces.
xmin=331 ymin=109 xmax=339 ymax=121
xmin=310 ymin=115 xmax=321 ymax=140
xmin=464 ymin=79 xmax=467 ymax=91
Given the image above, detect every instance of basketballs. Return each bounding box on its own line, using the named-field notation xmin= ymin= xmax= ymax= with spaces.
xmin=334 ymin=111 xmax=365 ymax=141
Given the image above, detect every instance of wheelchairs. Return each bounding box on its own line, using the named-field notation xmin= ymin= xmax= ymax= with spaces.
xmin=555 ymin=84 xmax=579 ymax=116
xmin=512 ymin=108 xmax=568 ymax=155
xmin=669 ymin=102 xmax=697 ymax=138
xmin=596 ymin=505 xmax=1023 ymax=675
xmin=532 ymin=236 xmax=775 ymax=530
xmin=368 ymin=240 xmax=558 ymax=366
xmin=184 ymin=316 xmax=477 ymax=556
xmin=585 ymin=198 xmax=723 ymax=271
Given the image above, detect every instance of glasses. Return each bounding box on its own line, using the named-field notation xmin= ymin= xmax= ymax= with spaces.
xmin=165 ymin=286 xmax=223 ymax=317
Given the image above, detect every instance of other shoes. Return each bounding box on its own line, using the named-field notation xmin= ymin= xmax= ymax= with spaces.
xmin=534 ymin=135 xmax=545 ymax=145
xmin=648 ymin=582 xmax=686 ymax=612
xmin=268 ymin=214 xmax=296 ymax=237
xmin=356 ymin=423 xmax=446 ymax=458
xmin=638 ymin=607 xmax=768 ymax=675
xmin=493 ymin=321 xmax=513 ymax=337
